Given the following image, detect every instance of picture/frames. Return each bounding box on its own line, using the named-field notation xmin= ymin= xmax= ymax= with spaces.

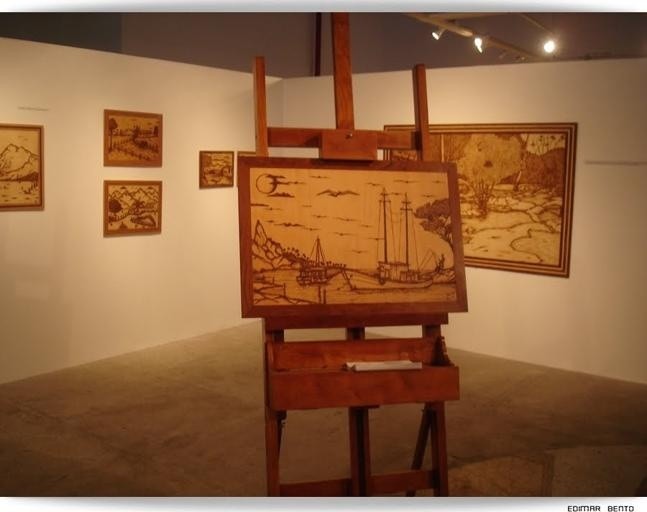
xmin=1 ymin=122 xmax=46 ymax=212
xmin=104 ymin=179 xmax=164 ymax=236
xmin=103 ymin=108 xmax=164 ymax=168
xmin=237 ymin=154 xmax=470 ymax=318
xmin=383 ymin=122 xmax=581 ymax=279
xmin=199 ymin=151 xmax=235 ymax=188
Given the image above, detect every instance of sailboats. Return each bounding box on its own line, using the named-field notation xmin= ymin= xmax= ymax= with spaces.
xmin=296 ymin=234 xmax=342 ymax=286
xmin=341 ymin=186 xmax=433 ymax=291
